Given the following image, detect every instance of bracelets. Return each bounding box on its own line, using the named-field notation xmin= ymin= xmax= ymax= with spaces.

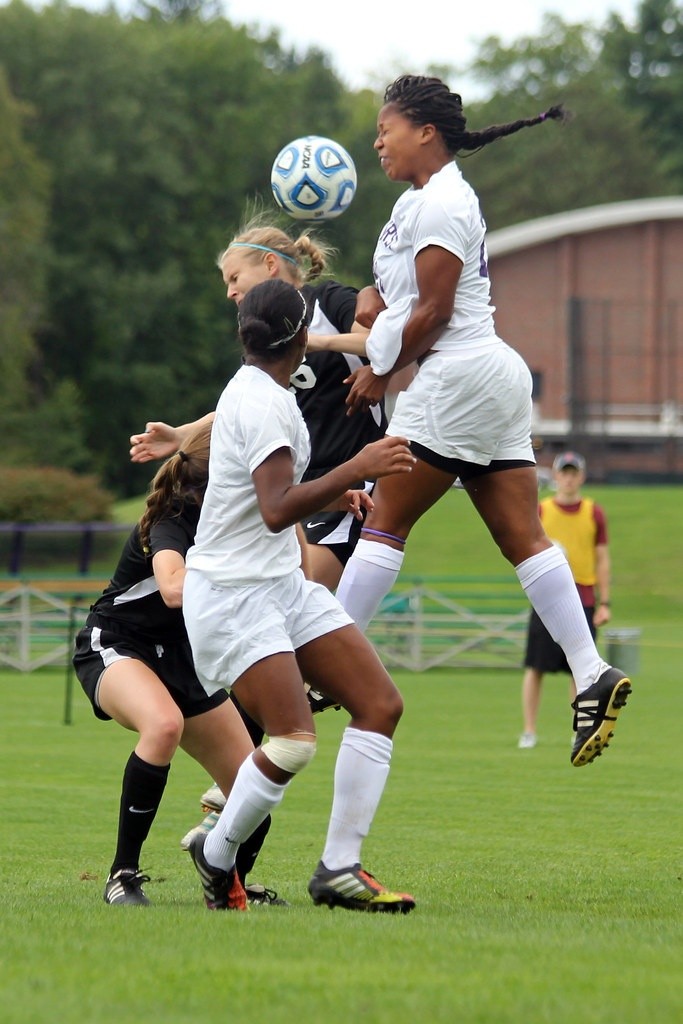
xmin=601 ymin=602 xmax=609 ymax=606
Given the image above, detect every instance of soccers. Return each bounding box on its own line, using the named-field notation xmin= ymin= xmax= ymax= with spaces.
xmin=269 ymin=135 xmax=358 ymax=226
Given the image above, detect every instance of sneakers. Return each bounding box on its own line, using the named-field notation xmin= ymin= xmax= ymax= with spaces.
xmin=243 ymin=883 xmax=290 ymax=909
xmin=189 ymin=834 xmax=250 ymax=913
xmin=570 ymin=667 xmax=634 ymax=768
xmin=103 ymin=867 xmax=160 ymax=908
xmin=301 ymin=683 xmax=346 ymax=717
xmin=308 ymin=861 xmax=416 ymax=913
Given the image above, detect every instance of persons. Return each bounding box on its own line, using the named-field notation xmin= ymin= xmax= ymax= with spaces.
xmin=516 ymin=452 xmax=611 ymax=751
xmin=308 ymin=75 xmax=632 ymax=767
xmin=180 ymin=279 xmax=415 ymax=914
xmin=74 ymin=421 xmax=289 ymax=909
xmin=129 ymin=228 xmax=388 ymax=847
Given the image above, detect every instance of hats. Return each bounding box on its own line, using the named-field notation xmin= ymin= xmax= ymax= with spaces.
xmin=553 ymin=451 xmax=593 ymax=476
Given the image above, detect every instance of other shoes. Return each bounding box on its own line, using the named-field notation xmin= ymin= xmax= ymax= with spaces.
xmin=519 ymin=733 xmax=541 ymax=750
xmin=182 ymin=811 xmax=233 ymax=853
xmin=200 ymin=781 xmax=228 ymax=812
